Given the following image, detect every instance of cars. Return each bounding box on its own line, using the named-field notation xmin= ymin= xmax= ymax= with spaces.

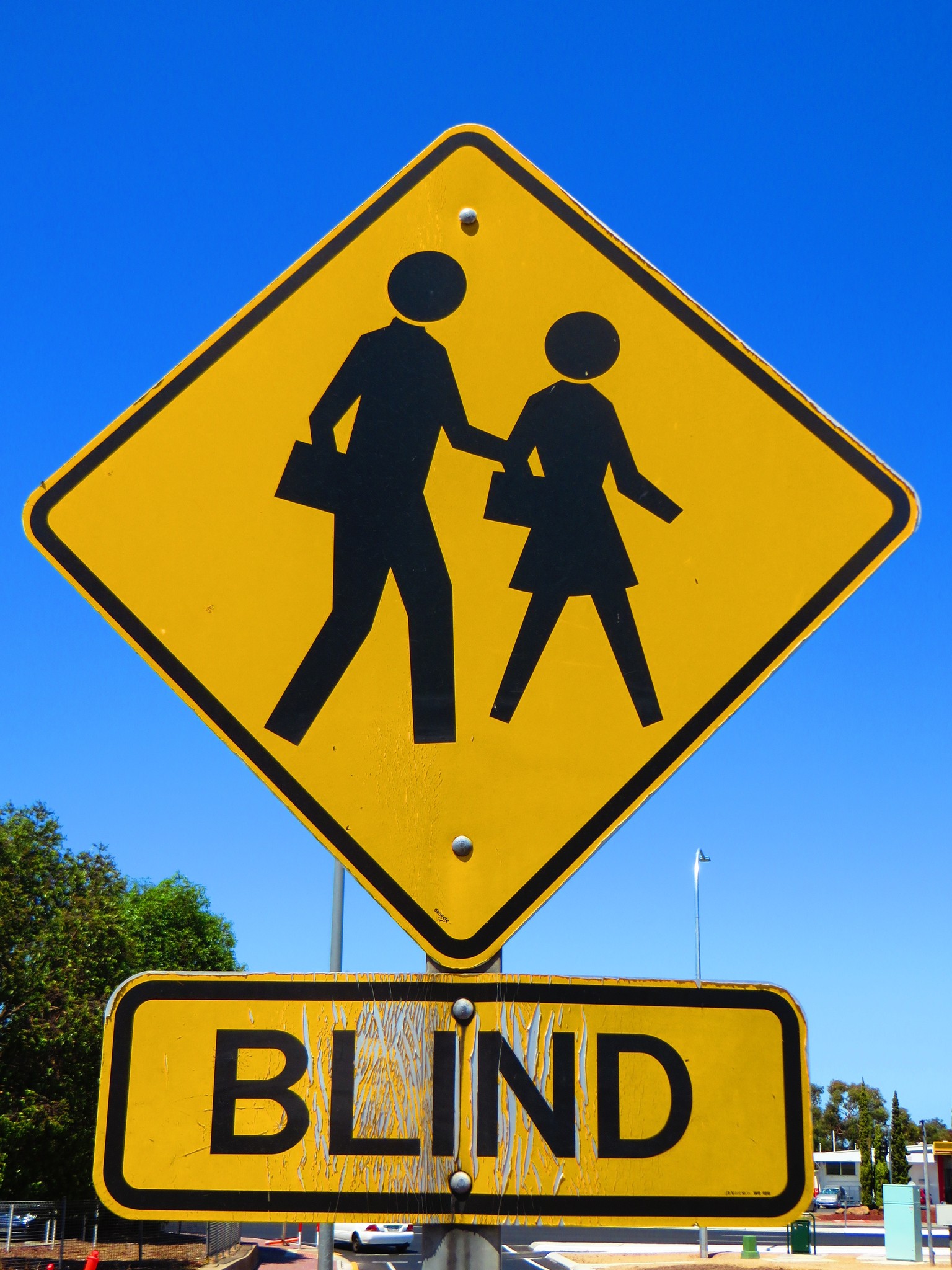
xmin=920 ymin=1187 xmax=933 ymax=1205
xmin=0 ymin=1201 xmax=64 ymax=1241
xmin=806 ymin=1197 xmax=817 ymax=1213
xmin=332 ymin=1222 xmax=415 ymax=1254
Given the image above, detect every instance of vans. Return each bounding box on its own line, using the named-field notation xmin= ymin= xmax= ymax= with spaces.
xmin=816 ymin=1185 xmax=847 ymax=1208
